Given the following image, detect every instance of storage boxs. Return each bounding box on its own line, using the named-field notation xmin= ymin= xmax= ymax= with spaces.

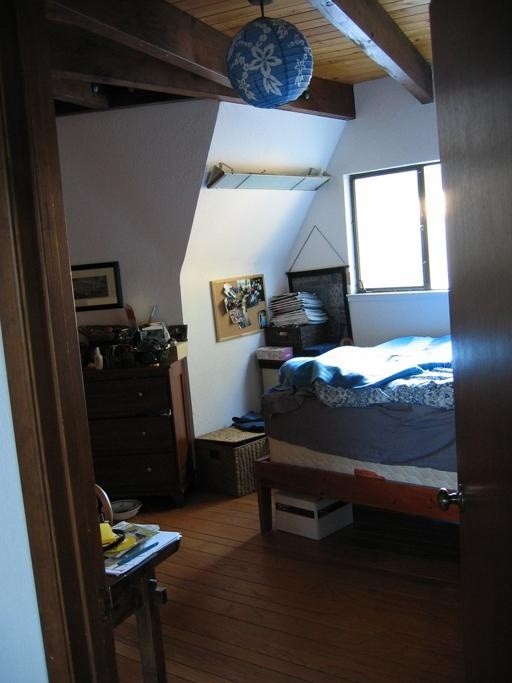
xmin=195 ymin=424 xmax=270 ymax=497
xmin=267 ymin=487 xmax=354 ymax=541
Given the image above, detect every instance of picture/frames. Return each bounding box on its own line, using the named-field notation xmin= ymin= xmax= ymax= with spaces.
xmin=70 ymin=260 xmax=124 ymax=312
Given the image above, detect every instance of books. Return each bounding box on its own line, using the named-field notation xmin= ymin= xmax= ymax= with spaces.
xmin=268 ymin=289 xmax=328 ymax=324
xmin=100 ymin=519 xmax=181 ymax=576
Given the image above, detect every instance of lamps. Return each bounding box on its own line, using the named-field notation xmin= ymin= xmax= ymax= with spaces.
xmin=224 ymin=0 xmax=315 ymax=109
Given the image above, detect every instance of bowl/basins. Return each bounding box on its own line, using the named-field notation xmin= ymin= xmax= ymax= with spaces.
xmin=78 ymin=324 xmax=130 ymax=344
xmin=100 ymin=499 xmax=143 ymax=520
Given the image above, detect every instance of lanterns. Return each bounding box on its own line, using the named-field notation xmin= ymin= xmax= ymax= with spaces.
xmin=225 ymin=16 xmax=315 ymax=110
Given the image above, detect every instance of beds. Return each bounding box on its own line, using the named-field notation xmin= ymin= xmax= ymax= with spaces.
xmin=252 ymin=344 xmax=461 ymax=540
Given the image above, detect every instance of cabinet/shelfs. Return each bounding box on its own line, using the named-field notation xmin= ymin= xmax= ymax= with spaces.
xmin=83 ymin=356 xmax=193 ymax=511
